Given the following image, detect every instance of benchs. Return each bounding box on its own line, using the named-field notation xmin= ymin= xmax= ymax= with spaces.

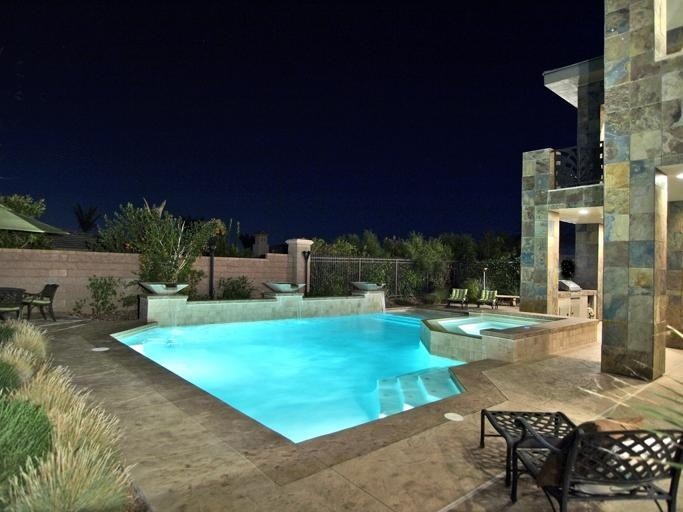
xmin=446 ymin=288 xmax=469 ymax=307
xmin=476 ymin=290 xmax=498 ymax=309
xmin=495 ymin=293 xmax=520 ymax=306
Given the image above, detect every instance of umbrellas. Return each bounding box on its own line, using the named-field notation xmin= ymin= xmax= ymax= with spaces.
xmin=0 ymin=203 xmax=71 ymax=236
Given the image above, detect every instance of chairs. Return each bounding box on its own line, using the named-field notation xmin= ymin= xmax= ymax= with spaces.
xmin=1 ymin=284 xmax=59 ymax=323
xmin=477 ymin=401 xmax=682 ymax=512
